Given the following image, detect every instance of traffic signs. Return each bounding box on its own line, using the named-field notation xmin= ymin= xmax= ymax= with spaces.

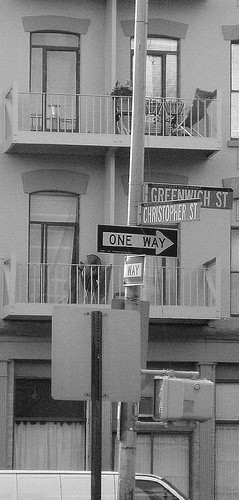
xmin=140 ymin=198 xmax=202 ymax=223
xmin=96 ymin=223 xmax=180 ymax=259
xmin=143 ymin=183 xmax=234 ymax=210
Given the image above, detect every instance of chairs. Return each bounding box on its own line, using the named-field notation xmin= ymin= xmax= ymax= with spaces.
xmin=170 ymin=87 xmax=219 ymax=137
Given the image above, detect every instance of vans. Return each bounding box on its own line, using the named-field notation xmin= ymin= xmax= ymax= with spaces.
xmin=0 ymin=467 xmax=187 ymax=500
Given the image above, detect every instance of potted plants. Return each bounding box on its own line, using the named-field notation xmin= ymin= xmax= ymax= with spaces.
xmin=145 ymin=100 xmax=162 ymax=116
xmin=107 ymin=79 xmax=134 ymax=113
xmin=163 ymin=100 xmax=185 ymax=115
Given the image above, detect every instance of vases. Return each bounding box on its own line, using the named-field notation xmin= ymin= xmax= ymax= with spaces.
xmin=49 ymin=104 xmax=61 ymax=116
